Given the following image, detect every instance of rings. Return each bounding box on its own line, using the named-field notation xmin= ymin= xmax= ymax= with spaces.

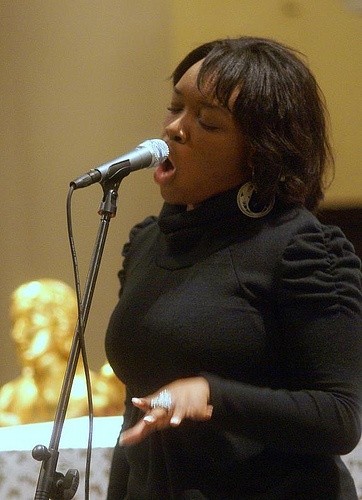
xmin=147 ymin=389 xmax=173 ymax=415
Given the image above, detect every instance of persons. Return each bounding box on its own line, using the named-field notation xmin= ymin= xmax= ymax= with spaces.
xmin=2 ymin=278 xmax=124 ymax=422
xmin=103 ymin=36 xmax=361 ymax=500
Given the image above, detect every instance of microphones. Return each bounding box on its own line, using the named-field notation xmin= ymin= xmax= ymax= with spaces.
xmin=68 ymin=137 xmax=172 ymax=191
